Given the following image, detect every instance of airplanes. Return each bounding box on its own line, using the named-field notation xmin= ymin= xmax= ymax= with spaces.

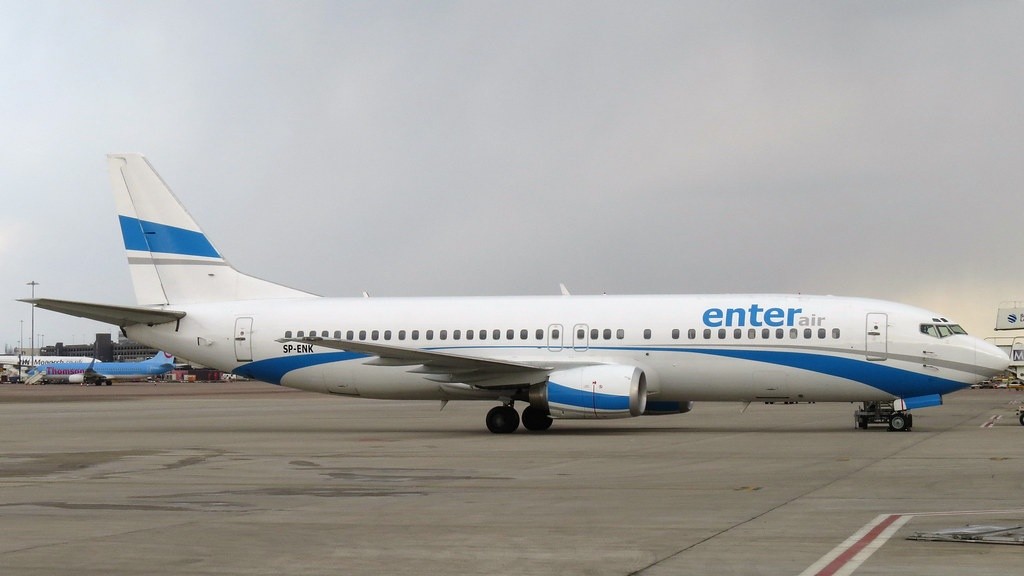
xmin=15 ymin=153 xmax=1010 ymax=433
xmin=27 ymin=351 xmax=176 ymax=386
xmin=0 ymin=356 xmax=102 ymax=385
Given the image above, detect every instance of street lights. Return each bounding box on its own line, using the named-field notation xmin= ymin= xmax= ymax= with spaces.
xmin=26 ymin=281 xmax=40 ymax=365
xmin=20 ymin=320 xmax=24 ymax=354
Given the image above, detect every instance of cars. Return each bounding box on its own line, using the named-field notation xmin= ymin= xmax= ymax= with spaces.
xmin=989 ymin=379 xmax=1007 ymax=388
xmin=971 ymin=381 xmax=990 ymax=389
